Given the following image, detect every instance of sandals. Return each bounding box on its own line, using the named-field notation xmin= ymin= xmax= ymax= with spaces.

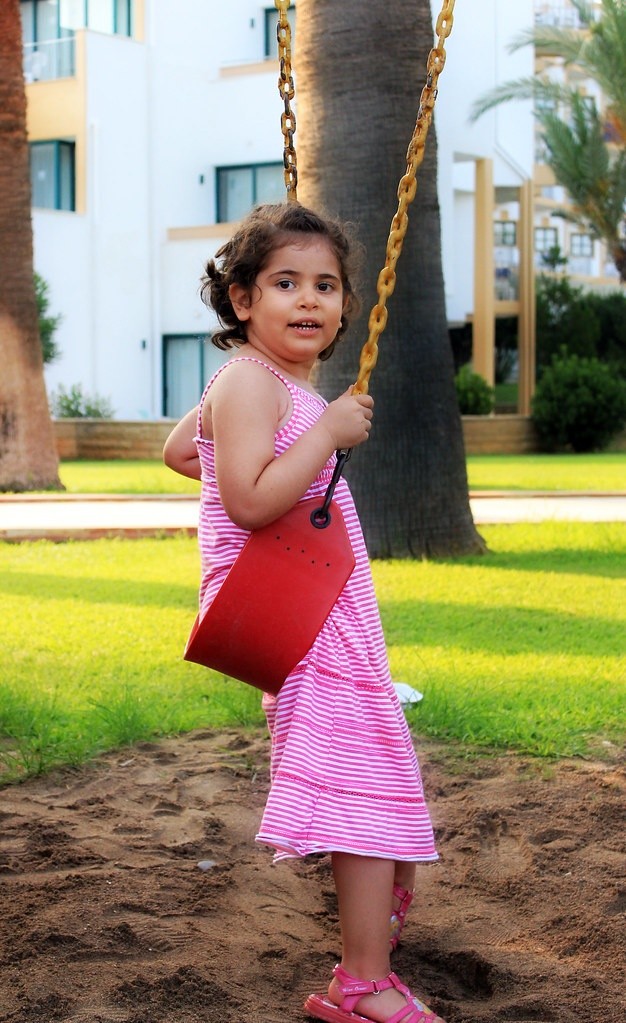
xmin=301 ymin=959 xmax=448 ymax=1023
xmin=377 ymin=875 xmax=420 ymax=956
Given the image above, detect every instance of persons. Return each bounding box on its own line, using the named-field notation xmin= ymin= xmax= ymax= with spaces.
xmin=158 ymin=202 xmax=457 ymax=1021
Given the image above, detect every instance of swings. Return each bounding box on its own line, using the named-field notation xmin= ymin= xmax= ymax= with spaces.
xmin=183 ymin=1 xmax=456 ymax=699
